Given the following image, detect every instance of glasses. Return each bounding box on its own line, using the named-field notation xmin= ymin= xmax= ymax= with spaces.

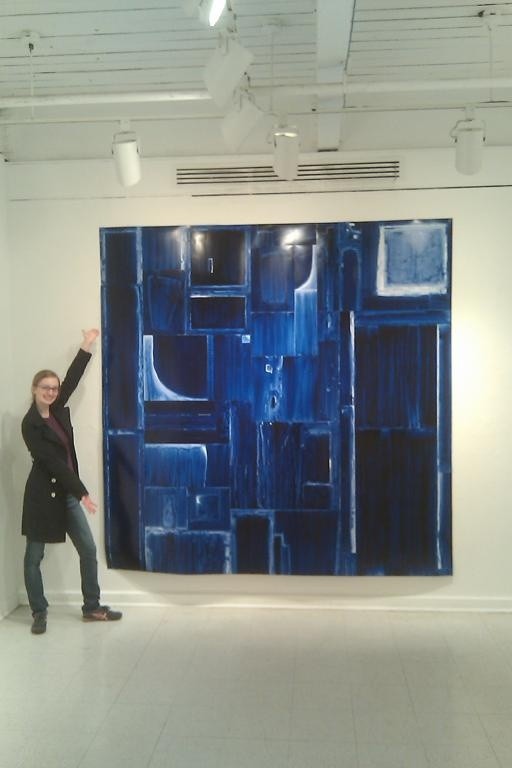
xmin=35 ymin=385 xmax=60 ymax=392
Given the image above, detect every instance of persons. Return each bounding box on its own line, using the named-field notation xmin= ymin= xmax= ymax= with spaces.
xmin=22 ymin=329 xmax=122 ymax=633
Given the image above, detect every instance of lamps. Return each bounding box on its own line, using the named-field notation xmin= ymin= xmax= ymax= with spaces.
xmin=109 ymin=117 xmax=144 ymax=188
xmin=184 ymin=0 xmax=266 ymax=154
xmin=270 ymin=101 xmax=490 ymax=180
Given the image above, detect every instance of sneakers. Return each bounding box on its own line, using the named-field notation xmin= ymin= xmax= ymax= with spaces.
xmin=31 ymin=612 xmax=47 ymax=635
xmin=82 ymin=607 xmax=122 ymax=622
